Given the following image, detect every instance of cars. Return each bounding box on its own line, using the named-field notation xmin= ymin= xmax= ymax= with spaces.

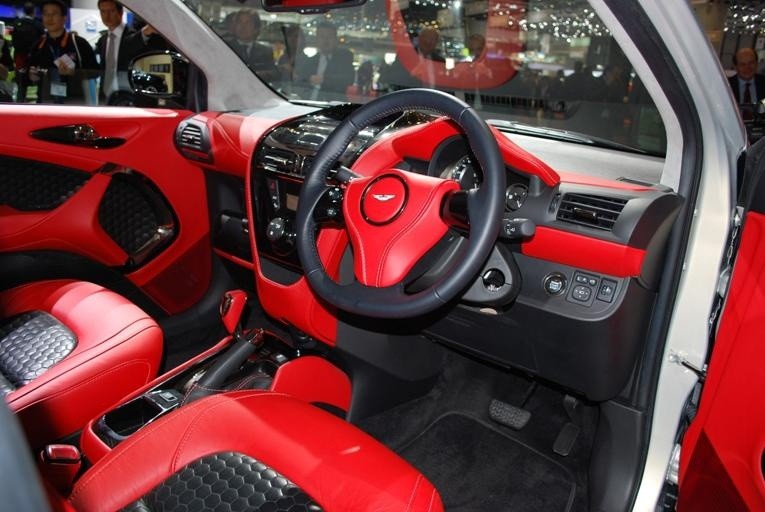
xmin=128 ymin=73 xmax=166 ymax=93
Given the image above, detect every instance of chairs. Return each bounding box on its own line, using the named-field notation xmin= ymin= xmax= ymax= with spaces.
xmin=2 ymin=278 xmax=164 ymax=448
xmin=2 ymin=390 xmax=445 ymax=512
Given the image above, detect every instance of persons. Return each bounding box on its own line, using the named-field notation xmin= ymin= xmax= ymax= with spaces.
xmin=0 ymin=0 xmax=764 ymax=152
